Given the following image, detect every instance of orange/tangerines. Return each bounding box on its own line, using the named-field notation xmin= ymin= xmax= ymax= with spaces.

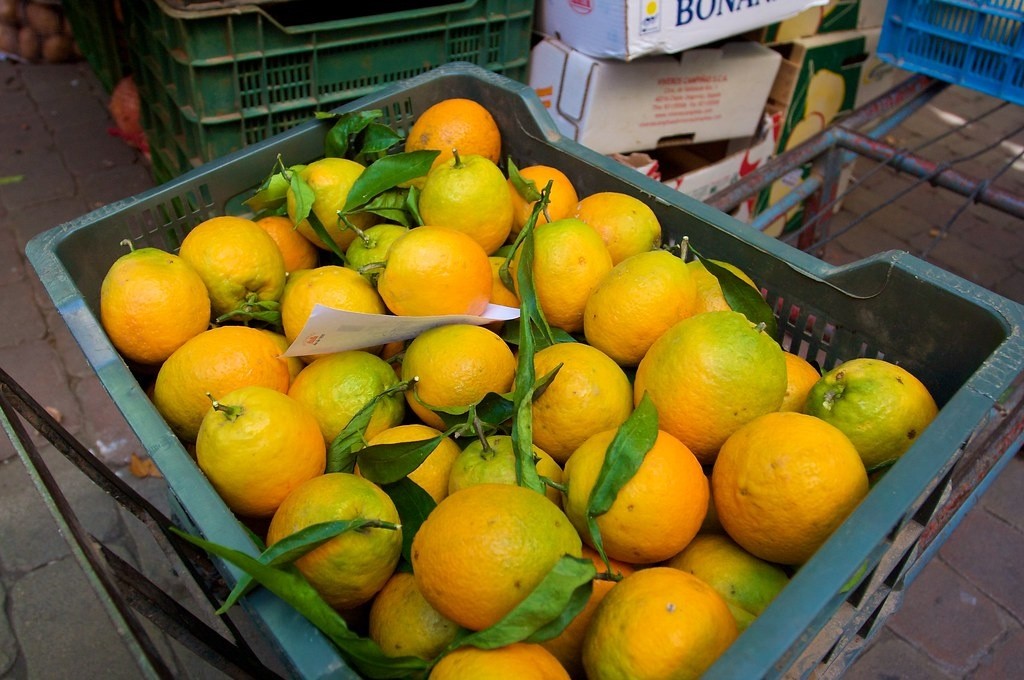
xmin=100 ymin=98 xmax=940 ymax=680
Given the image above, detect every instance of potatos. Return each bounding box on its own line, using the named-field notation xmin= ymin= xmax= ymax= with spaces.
xmin=0 ymin=0 xmax=87 ymax=62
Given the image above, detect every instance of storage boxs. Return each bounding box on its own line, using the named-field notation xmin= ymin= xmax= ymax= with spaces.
xmin=63 ymin=0 xmax=126 ymax=95
xmin=528 ymin=31 xmax=782 ymax=155
xmin=127 ymin=0 xmax=535 ymax=253
xmin=609 ymin=111 xmax=783 ymax=204
xmin=746 ymin=0 xmax=861 ymax=47
xmin=765 ymin=26 xmax=917 ymax=240
xmin=25 ymin=61 xmax=1024 ymax=678
xmin=875 ymin=0 xmax=1024 ymax=108
xmin=543 ymin=0 xmax=830 ymax=61
